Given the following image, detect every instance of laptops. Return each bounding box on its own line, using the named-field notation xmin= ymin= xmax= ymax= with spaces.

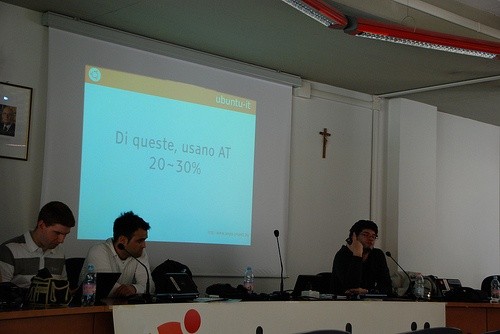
xmin=38 ymin=273 xmax=122 ymax=306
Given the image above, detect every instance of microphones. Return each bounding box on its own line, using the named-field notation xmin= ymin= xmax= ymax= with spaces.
xmin=269 ymin=230 xmax=290 ymax=301
xmin=384 ymin=250 xmax=414 ymax=300
xmin=117 ymin=242 xmax=156 ymax=304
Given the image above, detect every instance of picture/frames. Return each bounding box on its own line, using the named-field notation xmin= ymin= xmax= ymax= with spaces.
xmin=0 ymin=81 xmax=33 ymax=161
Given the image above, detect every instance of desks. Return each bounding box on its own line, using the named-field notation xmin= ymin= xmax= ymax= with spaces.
xmin=0 ymin=297 xmax=500 ymax=334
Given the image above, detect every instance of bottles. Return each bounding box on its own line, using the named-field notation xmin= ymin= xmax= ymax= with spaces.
xmin=244 ymin=268 xmax=254 ymax=294
xmin=490 ymin=276 xmax=500 ymax=303
xmin=81 ymin=264 xmax=96 ymax=306
xmin=415 ymin=273 xmax=424 ymax=298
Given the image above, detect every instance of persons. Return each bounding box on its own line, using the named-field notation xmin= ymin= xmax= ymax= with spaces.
xmin=0 ymin=201 xmax=76 ymax=309
xmin=78 ymin=211 xmax=156 ymax=296
xmin=332 ymin=220 xmax=393 ymax=297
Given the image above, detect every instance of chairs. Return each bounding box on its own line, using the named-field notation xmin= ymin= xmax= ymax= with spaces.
xmin=291 ymin=272 xmax=333 ymax=294
xmin=64 ymin=258 xmax=86 ymax=291
xmin=150 ymin=259 xmax=200 ymax=293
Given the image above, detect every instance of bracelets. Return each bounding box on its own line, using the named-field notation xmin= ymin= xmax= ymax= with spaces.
xmin=366 ymin=290 xmax=369 ymax=294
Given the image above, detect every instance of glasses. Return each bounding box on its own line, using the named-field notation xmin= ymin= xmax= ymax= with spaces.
xmin=360 ymin=231 xmax=379 ymax=240
